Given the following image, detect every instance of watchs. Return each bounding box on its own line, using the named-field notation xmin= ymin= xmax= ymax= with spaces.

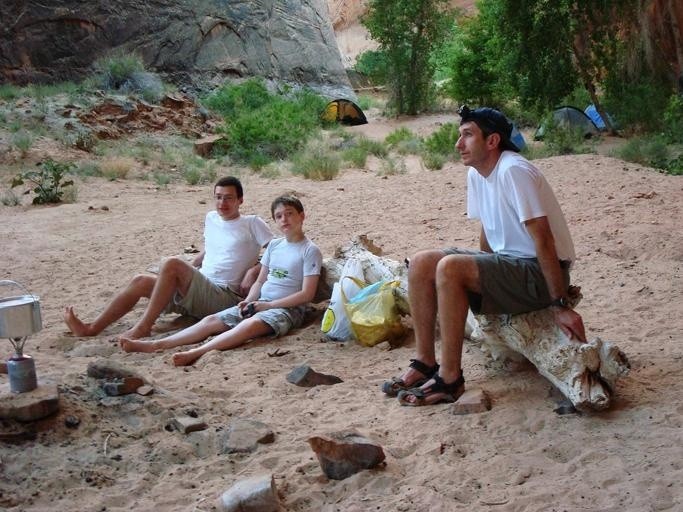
xmin=548 ymin=296 xmax=569 ymax=308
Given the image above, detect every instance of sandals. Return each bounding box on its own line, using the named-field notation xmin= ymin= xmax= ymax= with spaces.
xmin=397 ymin=369 xmax=465 ymax=406
xmin=381 ymin=359 xmax=439 ymax=395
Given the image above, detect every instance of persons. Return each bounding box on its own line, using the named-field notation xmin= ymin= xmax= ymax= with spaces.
xmin=380 ymin=101 xmax=590 ymax=407
xmin=118 ymin=196 xmax=322 ymax=367
xmin=61 ymin=176 xmax=278 ymax=343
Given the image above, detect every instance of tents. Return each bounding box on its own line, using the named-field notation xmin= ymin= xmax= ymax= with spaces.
xmin=583 ymin=102 xmax=615 ymax=132
xmin=531 ymin=105 xmax=601 ymax=141
xmin=317 ymin=98 xmax=368 ymax=131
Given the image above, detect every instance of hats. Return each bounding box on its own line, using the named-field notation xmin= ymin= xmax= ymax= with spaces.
xmin=455 ymin=104 xmax=520 ymax=153
